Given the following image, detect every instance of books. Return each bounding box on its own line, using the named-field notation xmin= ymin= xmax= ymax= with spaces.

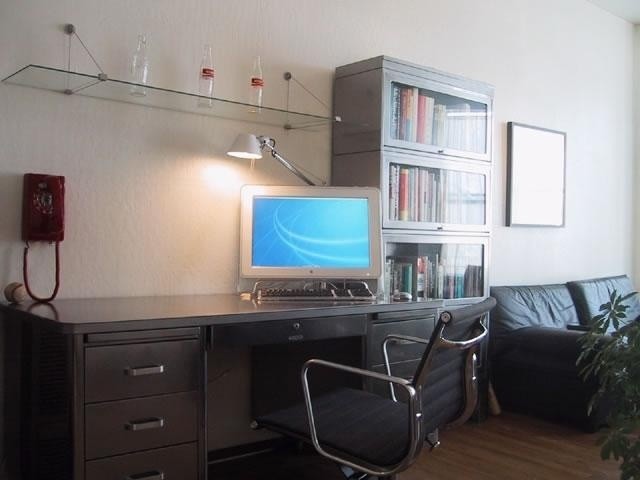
xmin=381 ymin=82 xmax=486 ymax=306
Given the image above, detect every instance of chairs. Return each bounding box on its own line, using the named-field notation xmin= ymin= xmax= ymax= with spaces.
xmin=249 ymin=296 xmax=497 ymax=480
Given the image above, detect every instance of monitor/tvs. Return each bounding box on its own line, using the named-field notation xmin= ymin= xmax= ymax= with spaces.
xmin=240 ymin=184 xmax=381 ymax=288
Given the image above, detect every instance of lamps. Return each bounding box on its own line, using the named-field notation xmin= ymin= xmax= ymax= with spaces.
xmin=227 ymin=133 xmax=326 ymax=186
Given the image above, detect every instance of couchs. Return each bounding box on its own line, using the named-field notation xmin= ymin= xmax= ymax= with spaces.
xmin=488 ymin=274 xmax=640 ymax=431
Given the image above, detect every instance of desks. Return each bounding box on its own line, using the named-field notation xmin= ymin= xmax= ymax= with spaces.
xmin=1 ymin=289 xmax=444 ymax=480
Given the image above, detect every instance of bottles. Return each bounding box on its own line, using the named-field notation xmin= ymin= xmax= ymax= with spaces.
xmin=248 ymin=54 xmax=263 ymax=113
xmin=197 ymin=44 xmax=214 ymax=107
xmin=130 ymin=33 xmax=149 ymax=97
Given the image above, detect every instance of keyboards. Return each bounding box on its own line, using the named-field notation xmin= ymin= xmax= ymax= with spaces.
xmin=257 ymin=287 xmax=375 ymax=300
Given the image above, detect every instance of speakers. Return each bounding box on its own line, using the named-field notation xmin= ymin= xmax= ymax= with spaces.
xmin=335 ymin=282 xmax=367 ymax=288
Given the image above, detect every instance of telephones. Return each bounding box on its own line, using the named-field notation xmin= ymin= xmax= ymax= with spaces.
xmin=21 ymin=173 xmax=65 ymax=241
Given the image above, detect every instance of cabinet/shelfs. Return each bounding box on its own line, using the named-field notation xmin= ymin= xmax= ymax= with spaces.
xmin=332 ymin=54 xmax=496 ymax=368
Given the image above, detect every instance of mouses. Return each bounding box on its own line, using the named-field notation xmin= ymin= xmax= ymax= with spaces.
xmin=393 ymin=292 xmax=412 ymax=301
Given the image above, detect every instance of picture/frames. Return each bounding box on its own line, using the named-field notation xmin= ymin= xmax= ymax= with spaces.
xmin=508 ymin=121 xmax=566 ymax=228
